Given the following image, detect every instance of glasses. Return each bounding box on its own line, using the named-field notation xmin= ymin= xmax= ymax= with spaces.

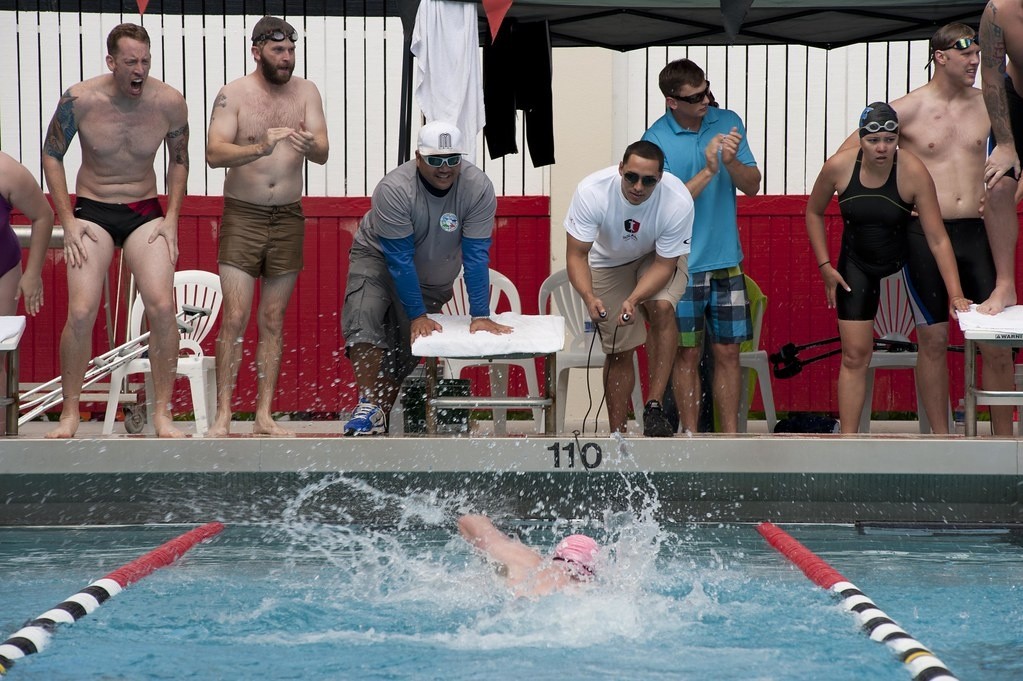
xmin=254 ymin=28 xmax=299 ymax=43
xmin=941 ymin=34 xmax=980 ymax=51
xmin=668 ymin=80 xmax=710 ymax=104
xmin=420 ymin=154 xmax=463 ymax=168
xmin=621 ymin=171 xmax=661 ymax=187
xmin=860 ymin=120 xmax=899 ymax=135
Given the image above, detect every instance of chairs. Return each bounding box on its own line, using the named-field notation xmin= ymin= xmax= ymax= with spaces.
xmin=102 ymin=270 xmax=224 ymax=434
xmin=439 ymin=265 xmax=543 ymax=436
xmin=677 ymin=275 xmax=778 ymax=434
xmin=538 ymin=268 xmax=644 ymax=435
xmin=858 ymin=270 xmax=956 ymax=434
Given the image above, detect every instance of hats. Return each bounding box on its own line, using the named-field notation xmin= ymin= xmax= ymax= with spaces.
xmin=859 ymin=102 xmax=899 ymax=140
xmin=416 ymin=120 xmax=468 ymax=156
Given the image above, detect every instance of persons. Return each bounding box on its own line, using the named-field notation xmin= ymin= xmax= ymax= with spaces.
xmin=639 ymin=59 xmax=761 ymax=432
xmin=203 ymin=16 xmax=329 ymax=436
xmin=564 ymin=140 xmax=695 ymax=438
xmin=458 ymin=515 xmax=599 ymax=591
xmin=977 ymin=0 xmax=1023 ymax=316
xmin=42 ymin=23 xmax=189 ymax=439
xmin=340 ymin=122 xmax=515 ymax=436
xmin=837 ymin=22 xmax=1015 ymax=436
xmin=805 ymin=101 xmax=974 ymax=434
xmin=0 ymin=151 xmax=54 ymax=435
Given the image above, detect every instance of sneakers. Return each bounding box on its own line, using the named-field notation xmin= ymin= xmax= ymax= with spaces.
xmin=642 ymin=399 xmax=673 ymax=437
xmin=343 ymin=398 xmax=386 ymax=437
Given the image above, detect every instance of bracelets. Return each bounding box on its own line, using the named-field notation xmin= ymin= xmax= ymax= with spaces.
xmin=471 ymin=316 xmax=488 ymax=321
xmin=819 ymin=261 xmax=830 ymax=268
